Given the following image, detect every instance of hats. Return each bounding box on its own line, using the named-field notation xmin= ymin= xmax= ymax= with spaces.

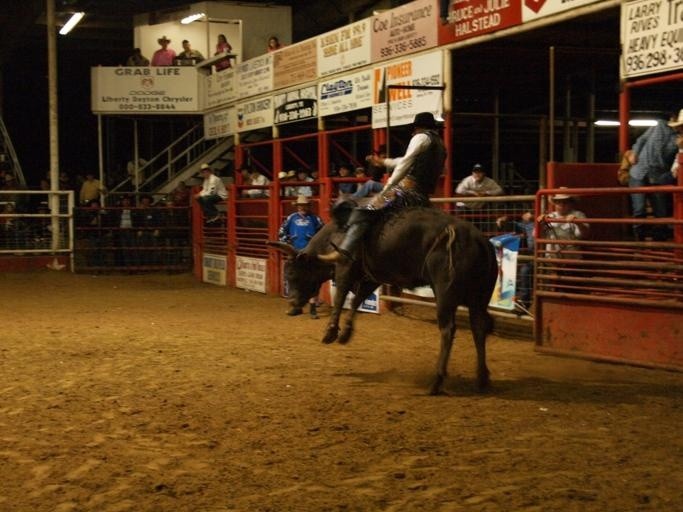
xmin=197 ymin=164 xmax=214 ymax=176
xmin=667 ymin=109 xmax=683 ymax=128
xmin=139 ymin=194 xmax=153 ymax=204
xmin=278 ymin=170 xmax=296 ymax=179
xmin=407 ymin=112 xmax=446 ymax=129
xmin=373 ymin=144 xmax=387 ymax=154
xmin=158 ymin=36 xmax=170 ymax=45
xmin=291 ymin=196 xmax=313 ymax=206
xmin=118 ymin=193 xmax=131 ymax=199
xmin=86 ymin=199 xmax=101 ymax=207
xmin=548 ymin=187 xmax=581 ymax=205
xmin=473 ymin=164 xmax=484 ymax=171
xmin=355 ymin=167 xmax=365 ymax=172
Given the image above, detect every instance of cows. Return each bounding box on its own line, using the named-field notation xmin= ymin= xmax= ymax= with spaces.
xmin=265 ymin=205 xmax=499 ymax=397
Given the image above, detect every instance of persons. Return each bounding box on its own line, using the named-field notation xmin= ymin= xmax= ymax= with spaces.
xmin=214 ymin=34 xmax=232 ymax=72
xmin=193 ymin=163 xmax=229 ymax=223
xmin=78 ymin=172 xmax=107 ymax=205
xmin=113 ymin=194 xmax=162 ymax=266
xmin=125 ymin=48 xmax=149 ymax=66
xmin=277 ymin=194 xmax=325 ymax=316
xmin=497 ymin=186 xmax=588 ymax=310
xmin=127 ymin=153 xmax=148 ymax=188
xmin=0 ymin=155 xmax=52 ymax=249
xmin=455 ymin=164 xmax=502 ymax=229
xmin=352 ymin=144 xmax=388 ymax=199
xmin=266 ymin=36 xmax=285 ymax=53
xmin=150 ymin=35 xmax=177 ymax=66
xmin=178 ymin=40 xmax=205 ymax=66
xmin=627 ymin=110 xmax=683 ymax=279
xmin=316 ymin=112 xmax=447 ymax=264
xmin=238 ymin=163 xmax=367 ymax=197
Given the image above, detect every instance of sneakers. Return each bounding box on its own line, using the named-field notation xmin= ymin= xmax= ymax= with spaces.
xmin=317 ymin=251 xmax=346 ymax=266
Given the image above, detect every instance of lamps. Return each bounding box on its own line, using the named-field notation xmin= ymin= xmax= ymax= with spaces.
xmin=55 ymin=9 xmax=85 ymax=35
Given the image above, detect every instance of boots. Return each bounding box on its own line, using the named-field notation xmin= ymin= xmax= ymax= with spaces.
xmin=286 ymin=308 xmax=303 ymax=315
xmin=310 ymin=303 xmax=318 ymax=318
